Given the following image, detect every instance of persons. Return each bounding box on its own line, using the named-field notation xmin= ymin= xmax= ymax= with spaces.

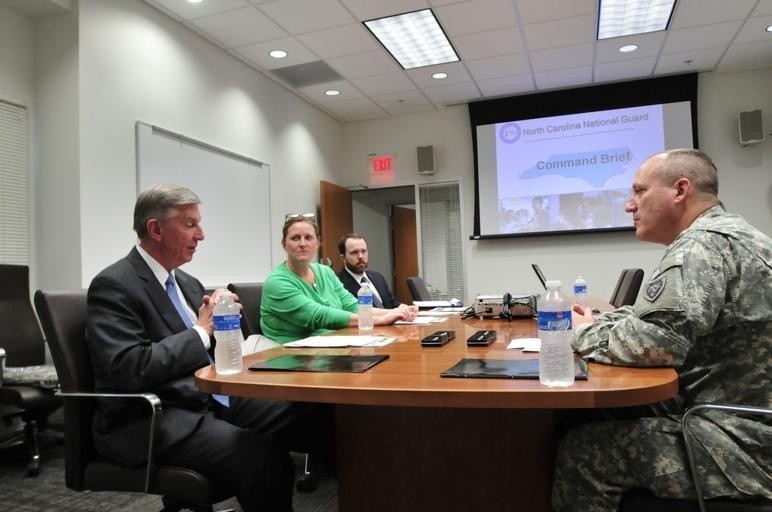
xmin=336 ymin=234 xmax=408 ymax=308
xmin=81 ymin=182 xmax=312 ymax=511
xmin=259 ymin=213 xmax=419 ymax=346
xmin=551 ymin=149 xmax=771 ymax=511
xmin=501 ymin=196 xmax=550 ymax=232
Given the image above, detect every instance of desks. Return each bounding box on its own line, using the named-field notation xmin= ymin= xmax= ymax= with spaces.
xmin=194 ymin=299 xmax=679 ymax=512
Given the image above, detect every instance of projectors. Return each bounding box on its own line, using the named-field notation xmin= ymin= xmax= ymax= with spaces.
xmin=473 ymin=294 xmax=539 ymax=321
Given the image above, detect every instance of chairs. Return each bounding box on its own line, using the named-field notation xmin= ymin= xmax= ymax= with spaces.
xmin=613 ymin=268 xmax=643 ymax=308
xmin=0 ymin=264 xmax=64 ymax=477
xmin=407 ymin=276 xmax=432 ymax=300
xmin=227 ymin=283 xmax=326 ymax=492
xmin=34 ymin=290 xmax=238 ymax=511
xmin=623 ymin=404 xmax=772 ymax=512
xmin=609 ymin=268 xmax=629 ymax=304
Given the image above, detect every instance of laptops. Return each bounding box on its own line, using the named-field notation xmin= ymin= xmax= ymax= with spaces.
xmin=531 ymin=263 xmax=551 ymax=290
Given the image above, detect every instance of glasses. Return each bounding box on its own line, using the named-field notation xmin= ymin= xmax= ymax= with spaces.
xmin=284 ymin=212 xmax=317 ymax=223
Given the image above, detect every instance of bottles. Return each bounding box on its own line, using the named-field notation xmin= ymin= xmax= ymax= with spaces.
xmin=574 ymin=273 xmax=588 ymax=308
xmin=357 ymin=282 xmax=374 ymax=331
xmin=212 ymin=279 xmax=243 ymax=375
xmin=538 ymin=280 xmax=575 ymax=388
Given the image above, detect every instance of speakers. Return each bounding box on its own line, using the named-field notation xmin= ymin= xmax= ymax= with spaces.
xmin=416 ymin=144 xmax=436 ymax=174
xmin=737 ymin=108 xmax=767 ymax=146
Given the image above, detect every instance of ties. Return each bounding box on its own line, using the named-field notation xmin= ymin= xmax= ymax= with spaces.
xmin=165 ymin=274 xmax=230 ymax=409
xmin=360 ymin=277 xmax=384 ymax=309
